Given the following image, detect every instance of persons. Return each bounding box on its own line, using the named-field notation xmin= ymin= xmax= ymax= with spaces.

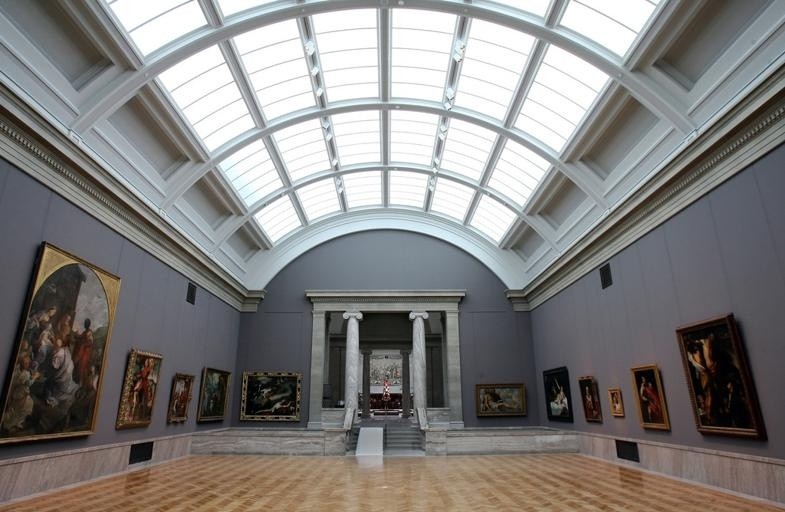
xmin=640 ymin=376 xmax=662 ymax=422
xmin=373 ymin=366 xmax=402 ymax=382
xmin=485 ymin=389 xmax=519 ymax=410
xmin=552 ymin=387 xmax=565 ymax=405
xmin=132 ymin=358 xmax=154 ymax=406
xmin=584 ymin=386 xmax=593 ymax=418
xmin=613 ymin=394 xmax=621 ymax=412
xmin=1 ymin=305 xmax=95 ymax=431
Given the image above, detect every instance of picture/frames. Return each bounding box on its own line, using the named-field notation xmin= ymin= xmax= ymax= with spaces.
xmin=1 ymin=241 xmax=123 ymax=445
xmin=541 ymin=311 xmax=770 ymax=444
xmin=197 ymin=364 xmax=233 ymax=424
xmin=114 ymin=346 xmax=164 ymax=431
xmin=239 ymin=369 xmax=302 ymax=422
xmin=475 ymin=382 xmax=528 ymax=418
xmin=167 ymin=371 xmax=196 ymax=424
